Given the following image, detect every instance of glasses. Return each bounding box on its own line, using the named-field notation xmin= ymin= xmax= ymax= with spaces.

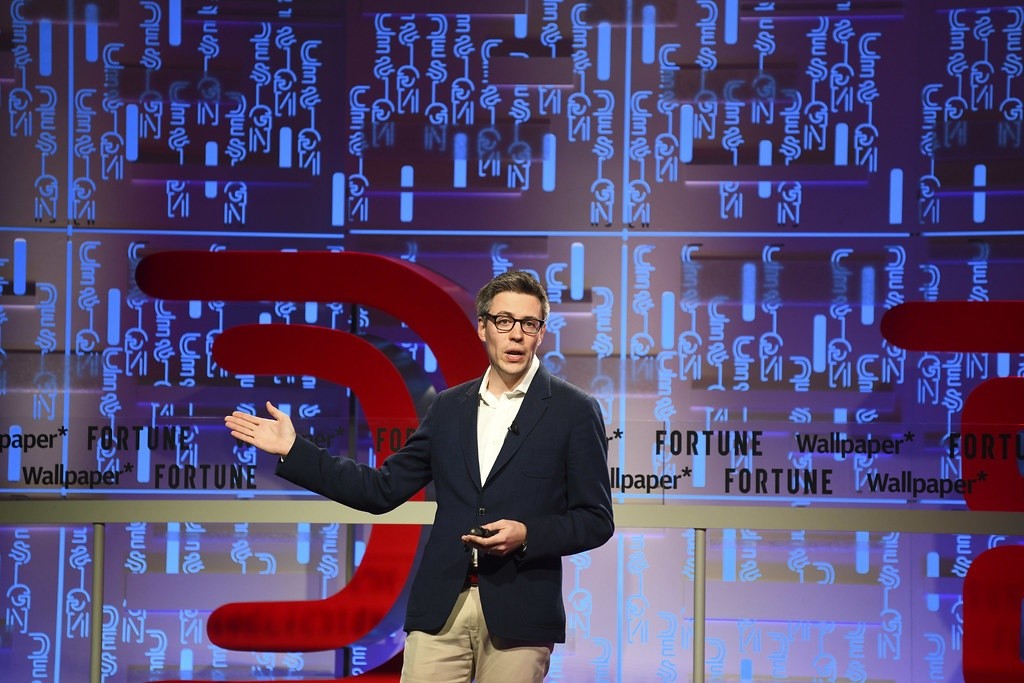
xmin=480 ymin=311 xmax=545 ymax=334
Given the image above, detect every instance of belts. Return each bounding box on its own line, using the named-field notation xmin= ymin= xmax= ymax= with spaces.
xmin=465 ymin=572 xmax=481 ymax=588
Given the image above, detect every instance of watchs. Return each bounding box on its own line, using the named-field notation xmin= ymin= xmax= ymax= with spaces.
xmin=520 ymin=532 xmax=528 ymax=554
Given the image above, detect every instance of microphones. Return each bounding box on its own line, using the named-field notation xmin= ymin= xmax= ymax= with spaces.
xmin=508 ymin=420 xmax=520 ymax=435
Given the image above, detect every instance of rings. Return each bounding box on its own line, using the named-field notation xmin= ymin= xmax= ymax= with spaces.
xmin=486 ymin=550 xmax=491 ymax=555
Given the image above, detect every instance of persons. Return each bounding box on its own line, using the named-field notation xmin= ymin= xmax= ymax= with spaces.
xmin=224 ymin=268 xmax=615 ymax=683
